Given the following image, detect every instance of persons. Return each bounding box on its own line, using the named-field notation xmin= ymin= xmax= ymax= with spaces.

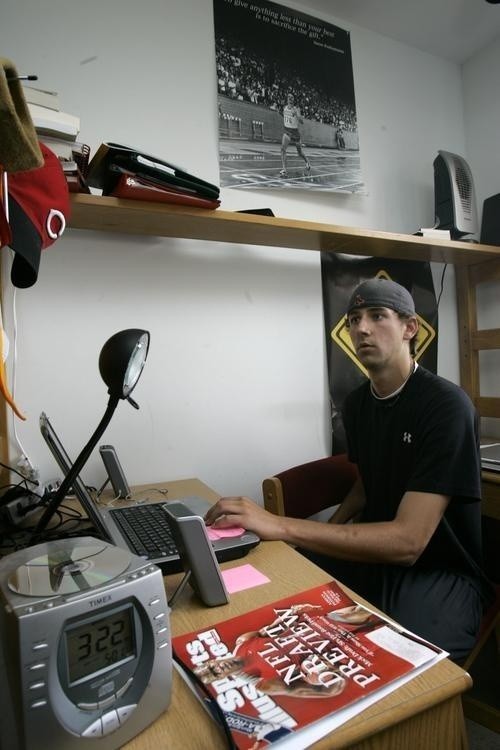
xmin=205 ymin=273 xmax=484 ymax=663
xmin=192 ymin=597 xmax=394 ymax=700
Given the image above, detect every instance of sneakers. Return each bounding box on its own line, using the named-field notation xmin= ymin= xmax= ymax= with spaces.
xmin=279 ymin=169 xmax=287 ymax=174
xmin=304 ymin=165 xmax=312 ymax=176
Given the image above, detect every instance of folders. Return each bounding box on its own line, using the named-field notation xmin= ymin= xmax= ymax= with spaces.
xmin=108 ymin=152 xmax=221 ymax=210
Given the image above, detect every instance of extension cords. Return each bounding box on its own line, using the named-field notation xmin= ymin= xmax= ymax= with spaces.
xmin=6 ymin=476 xmax=66 ymax=525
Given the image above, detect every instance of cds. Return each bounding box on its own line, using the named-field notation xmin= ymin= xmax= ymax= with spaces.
xmin=5 ymin=543 xmax=131 ymax=601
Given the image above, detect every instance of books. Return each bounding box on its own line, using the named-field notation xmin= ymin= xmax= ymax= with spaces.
xmin=169 ymin=580 xmax=449 ymax=748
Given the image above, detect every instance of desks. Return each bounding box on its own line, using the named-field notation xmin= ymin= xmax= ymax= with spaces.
xmin=1 ymin=476 xmax=473 ymax=748
xmin=460 ymin=437 xmax=500 ymax=732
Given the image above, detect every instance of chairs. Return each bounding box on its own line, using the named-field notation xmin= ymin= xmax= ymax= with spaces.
xmin=263 ymin=451 xmax=500 ymax=749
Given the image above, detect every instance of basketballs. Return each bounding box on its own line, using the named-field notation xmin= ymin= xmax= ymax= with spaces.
xmin=300 ymin=653 xmax=337 ymax=685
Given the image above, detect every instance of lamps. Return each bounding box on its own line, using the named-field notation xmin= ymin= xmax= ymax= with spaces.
xmin=29 ymin=327 xmax=151 ymax=546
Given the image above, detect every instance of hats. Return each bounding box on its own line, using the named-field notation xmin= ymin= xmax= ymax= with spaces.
xmin=347 ymin=278 xmax=415 ymax=318
xmin=0 ymin=56 xmax=71 ymax=289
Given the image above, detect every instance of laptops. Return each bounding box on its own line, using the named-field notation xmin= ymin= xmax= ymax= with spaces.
xmin=38 ymin=410 xmax=261 ymax=576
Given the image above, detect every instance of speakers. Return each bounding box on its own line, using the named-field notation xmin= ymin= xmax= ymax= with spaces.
xmin=431 ymin=147 xmax=481 ymax=244
xmin=161 ymin=497 xmax=230 ymax=611
xmin=98 ymin=441 xmax=133 ymax=500
